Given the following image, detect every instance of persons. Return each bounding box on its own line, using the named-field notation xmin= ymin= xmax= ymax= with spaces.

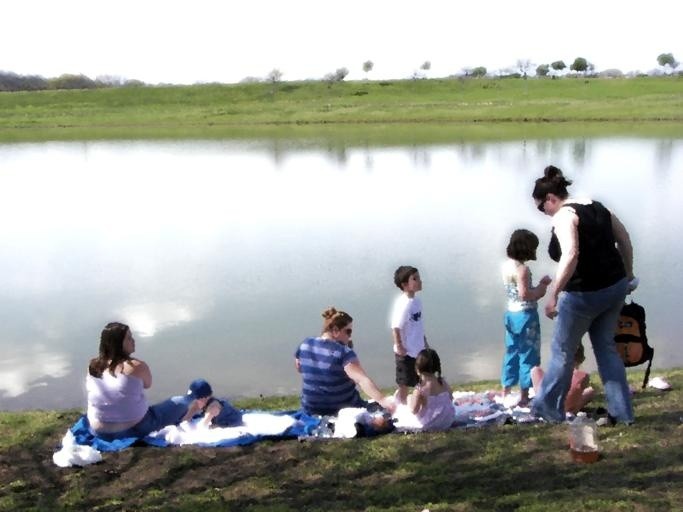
xmin=530 ymin=164 xmax=639 ymax=427
xmin=291 ymin=307 xmax=395 ymax=417
xmin=386 ymin=263 xmax=429 ymax=404
xmin=500 ymin=228 xmax=552 ymax=411
xmin=527 ymin=341 xmax=595 ymax=417
xmin=408 ymin=349 xmax=456 ymax=431
xmin=84 ymin=320 xmax=188 ymax=442
xmin=179 ymin=376 xmax=243 ymax=429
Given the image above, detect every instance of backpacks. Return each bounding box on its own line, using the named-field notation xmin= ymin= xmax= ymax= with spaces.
xmin=613 ymin=291 xmax=654 ymax=368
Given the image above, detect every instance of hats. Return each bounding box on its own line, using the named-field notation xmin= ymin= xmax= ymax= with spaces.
xmin=183 ymin=379 xmax=212 ymax=400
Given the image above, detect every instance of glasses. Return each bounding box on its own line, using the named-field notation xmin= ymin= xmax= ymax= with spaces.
xmin=537 ymin=198 xmax=548 ymax=212
xmin=338 ymin=328 xmax=352 ymax=336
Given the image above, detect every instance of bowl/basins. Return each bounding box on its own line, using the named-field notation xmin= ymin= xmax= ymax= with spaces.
xmin=570 ymin=448 xmax=600 ymax=463
